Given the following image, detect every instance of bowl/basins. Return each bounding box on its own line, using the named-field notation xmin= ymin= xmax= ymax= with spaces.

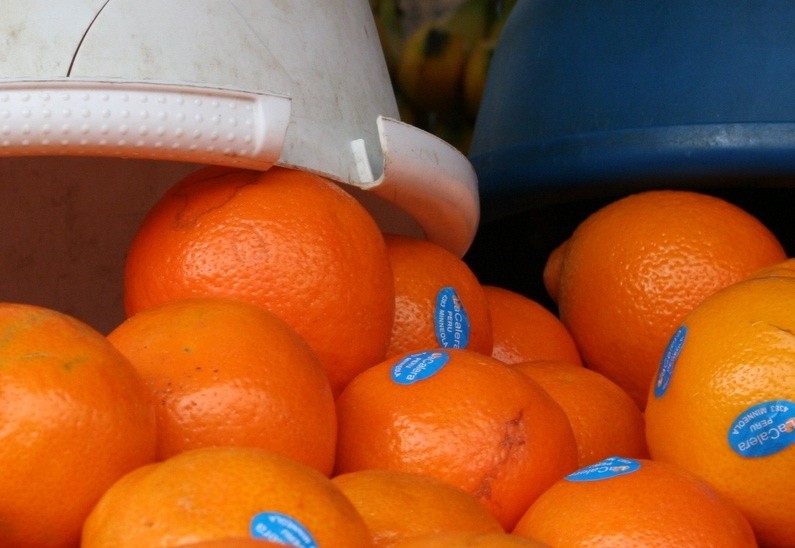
xmin=1 ymin=1 xmax=486 ymax=333
xmin=444 ymin=2 xmax=795 ymax=326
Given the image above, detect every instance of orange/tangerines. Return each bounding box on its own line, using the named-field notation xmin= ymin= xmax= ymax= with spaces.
xmin=0 ymin=165 xmax=795 ymax=548
